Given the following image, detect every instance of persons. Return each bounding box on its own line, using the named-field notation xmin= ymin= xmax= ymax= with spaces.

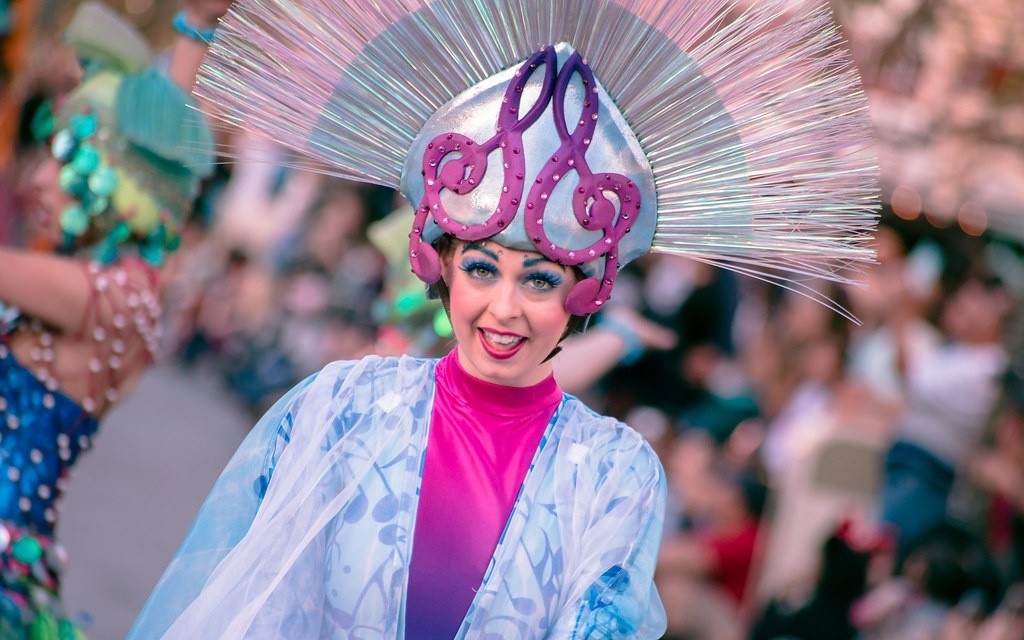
xmin=157 ymin=0 xmax=1024 ymax=640
xmin=122 ymin=39 xmax=668 ymax=640
xmin=0 ymin=68 xmax=208 ymax=640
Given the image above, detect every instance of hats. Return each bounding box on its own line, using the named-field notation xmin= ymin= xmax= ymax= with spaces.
xmin=180 ymin=0 xmax=884 ymax=328
xmin=46 ymin=70 xmax=212 ymax=253
